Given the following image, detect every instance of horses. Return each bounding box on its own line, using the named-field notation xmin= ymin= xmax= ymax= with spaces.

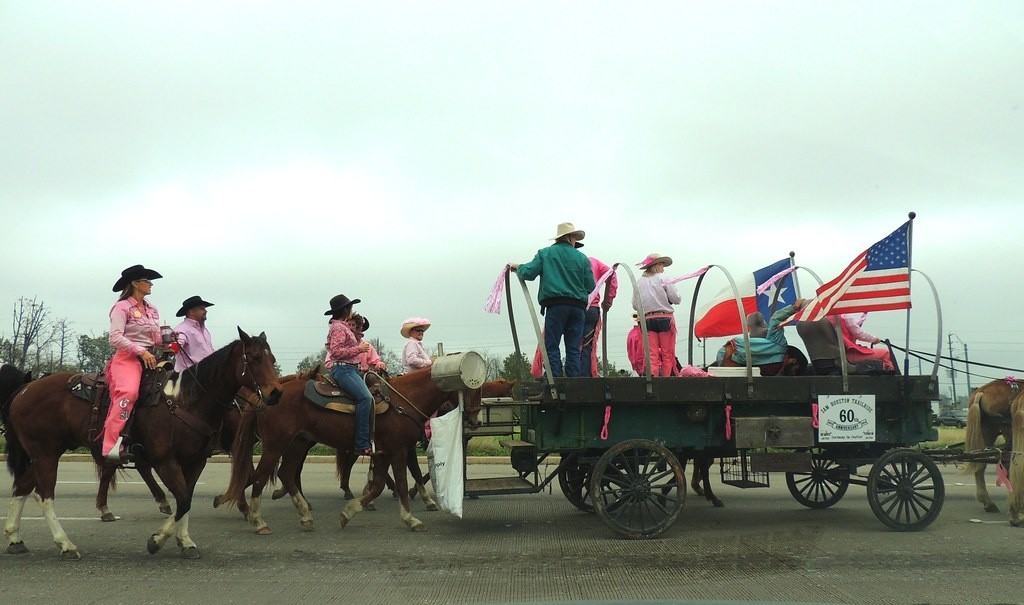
xmin=0 ymin=327 xmax=284 ymax=563
xmin=213 ymin=359 xmax=514 ymax=535
xmin=966 ymin=371 xmax=1024 ymax=526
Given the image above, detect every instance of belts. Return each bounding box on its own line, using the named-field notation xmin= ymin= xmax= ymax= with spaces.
xmin=646 ymin=311 xmax=670 ymax=316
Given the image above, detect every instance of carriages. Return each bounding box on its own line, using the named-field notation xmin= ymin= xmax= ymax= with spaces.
xmin=464 ymin=261 xmax=1024 ymax=541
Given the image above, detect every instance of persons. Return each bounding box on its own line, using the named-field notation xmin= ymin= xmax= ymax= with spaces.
xmin=325 ymin=314 xmax=386 ymax=373
xmin=511 ymin=221 xmax=595 ymax=378
xmin=717 ymin=299 xmax=808 ymax=377
xmin=747 ymin=311 xmax=768 ymax=338
xmin=825 ymin=312 xmax=895 ymax=373
xmin=168 ymin=296 xmax=215 ymax=372
xmin=102 ymin=266 xmax=163 ymax=460
xmin=400 ymin=318 xmax=438 ymax=440
xmin=580 ymin=256 xmax=617 ymax=378
xmin=632 ymin=254 xmax=680 ymax=375
xmin=628 ymin=317 xmax=646 ymax=373
xmin=326 ymin=295 xmax=381 ymax=457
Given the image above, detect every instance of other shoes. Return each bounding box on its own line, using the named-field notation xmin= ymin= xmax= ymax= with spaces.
xmin=354 ymin=447 xmax=383 ymax=456
xmin=104 ymin=449 xmax=135 ymax=461
xmin=528 ymin=392 xmax=544 ymax=401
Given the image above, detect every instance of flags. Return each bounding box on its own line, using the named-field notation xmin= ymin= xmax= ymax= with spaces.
xmin=773 ymin=220 xmax=913 ymax=329
xmin=754 ymin=257 xmax=801 ymax=326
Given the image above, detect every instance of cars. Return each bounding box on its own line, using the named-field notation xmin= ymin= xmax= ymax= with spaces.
xmin=937 ymin=409 xmax=967 ymax=428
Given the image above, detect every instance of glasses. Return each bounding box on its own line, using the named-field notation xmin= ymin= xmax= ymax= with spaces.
xmin=137 ymin=279 xmax=152 ymax=283
xmin=414 ymin=328 xmax=425 ymax=332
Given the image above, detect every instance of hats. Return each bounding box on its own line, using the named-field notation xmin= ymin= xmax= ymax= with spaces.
xmin=788 ymin=346 xmax=808 ymax=366
xmin=552 ymin=242 xmax=584 ymax=249
xmin=176 ymin=296 xmax=214 ymax=317
xmin=639 ymin=254 xmax=673 ymax=269
xmin=346 ymin=314 xmax=369 ymax=332
xmin=400 ymin=318 xmax=431 ymax=338
xmin=324 ymin=294 xmax=361 ymax=315
xmin=113 ymin=264 xmax=163 ymax=292
xmin=548 ymin=223 xmax=585 ymax=240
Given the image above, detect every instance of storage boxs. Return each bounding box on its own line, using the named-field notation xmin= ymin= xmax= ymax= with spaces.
xmin=464 ymin=397 xmax=513 ymax=434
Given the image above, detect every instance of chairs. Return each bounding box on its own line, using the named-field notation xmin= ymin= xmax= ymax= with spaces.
xmin=797 ymin=319 xmax=888 ymax=375
